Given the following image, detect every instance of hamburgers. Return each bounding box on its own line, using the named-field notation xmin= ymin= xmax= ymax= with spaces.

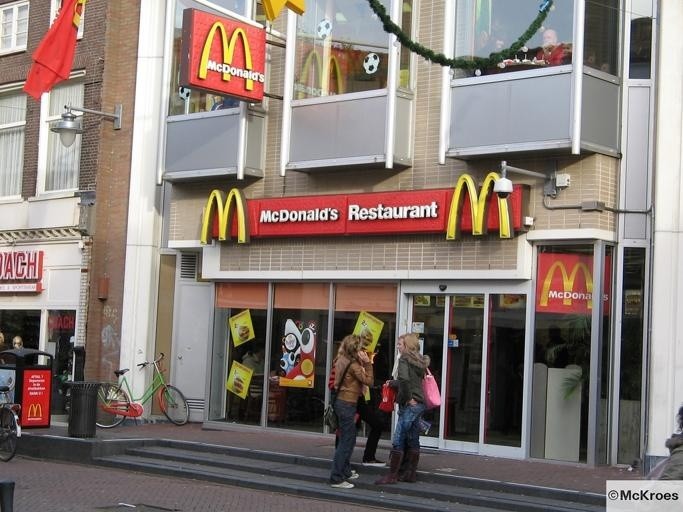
xmin=234 ymin=378 xmax=244 ymax=392
xmin=238 ymin=326 xmax=250 ymax=339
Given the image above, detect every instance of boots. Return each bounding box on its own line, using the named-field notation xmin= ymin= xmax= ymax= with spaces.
xmin=375 ymin=448 xmax=418 ymax=483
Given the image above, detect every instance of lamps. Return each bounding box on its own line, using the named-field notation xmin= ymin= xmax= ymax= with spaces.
xmin=51 ymin=104 xmax=123 ymax=147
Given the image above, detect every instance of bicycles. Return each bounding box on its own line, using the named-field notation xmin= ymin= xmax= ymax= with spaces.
xmin=0 ymin=386 xmax=23 ymax=462
xmin=95 ymin=352 xmax=190 ymax=428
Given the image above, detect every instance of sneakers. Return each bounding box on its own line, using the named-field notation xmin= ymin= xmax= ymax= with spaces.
xmin=362 ymin=460 xmax=386 ymax=466
xmin=331 ymin=471 xmax=359 ymax=489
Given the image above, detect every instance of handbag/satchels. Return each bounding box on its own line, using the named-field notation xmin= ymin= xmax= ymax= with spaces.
xmin=379 ymin=384 xmax=396 ymax=412
xmin=324 ymin=405 xmax=338 ymax=430
xmin=422 ymin=368 xmax=441 ymax=409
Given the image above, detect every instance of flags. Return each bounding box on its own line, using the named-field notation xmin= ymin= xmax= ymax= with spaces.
xmin=21 ymin=2 xmax=84 ymax=104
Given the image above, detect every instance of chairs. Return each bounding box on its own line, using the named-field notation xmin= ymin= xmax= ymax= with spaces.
xmin=227 ymin=372 xmax=264 ymax=421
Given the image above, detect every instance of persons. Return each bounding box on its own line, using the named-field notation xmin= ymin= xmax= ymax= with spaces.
xmin=535 ymin=29 xmax=567 ymax=67
xmin=326 ymin=333 xmax=373 ymax=491
xmin=374 ymin=332 xmax=431 ymax=486
xmin=12 ymin=335 xmax=23 ymax=350
xmin=488 ymin=38 xmax=505 ymax=55
xmin=355 ymin=344 xmax=391 ymax=468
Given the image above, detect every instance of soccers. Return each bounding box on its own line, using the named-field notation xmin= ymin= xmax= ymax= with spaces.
xmin=317 ymin=19 xmax=333 ymax=40
xmin=363 ymin=52 xmax=380 ymax=75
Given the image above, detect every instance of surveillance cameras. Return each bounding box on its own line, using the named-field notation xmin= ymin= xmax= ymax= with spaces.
xmin=492 ymin=178 xmax=514 ymax=199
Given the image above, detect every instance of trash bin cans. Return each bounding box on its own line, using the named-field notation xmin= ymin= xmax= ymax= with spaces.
xmin=0 ymin=348 xmax=53 ymax=429
xmin=64 ymin=381 xmax=103 ymax=438
